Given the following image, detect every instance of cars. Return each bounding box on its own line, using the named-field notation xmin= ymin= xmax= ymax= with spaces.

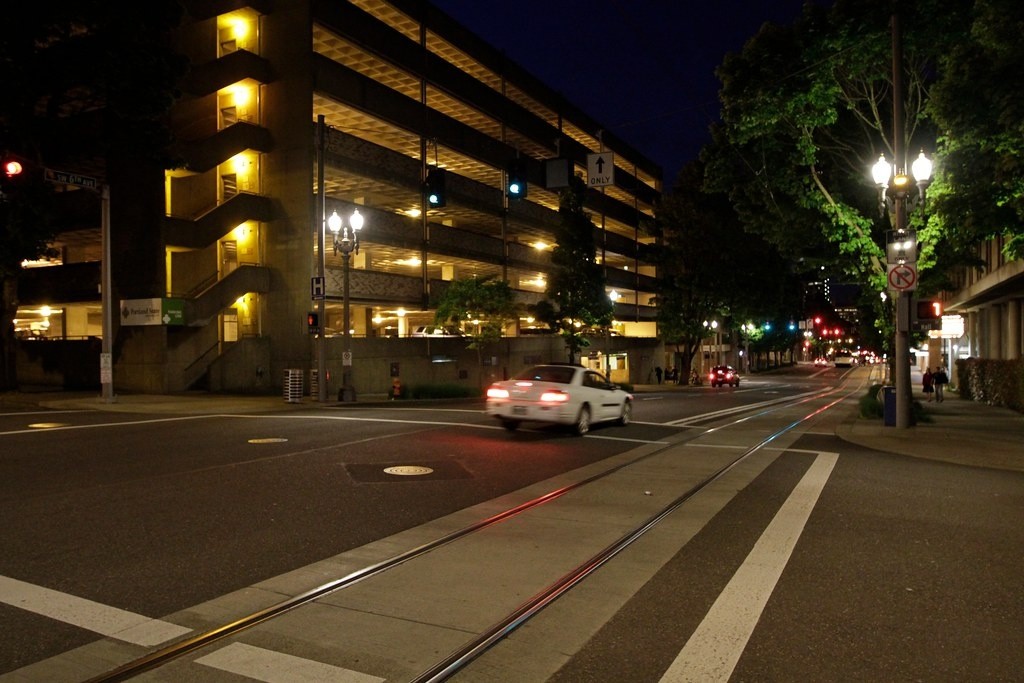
xmin=814 ymin=358 xmax=827 ymax=367
xmin=485 ymin=364 xmax=634 ymax=436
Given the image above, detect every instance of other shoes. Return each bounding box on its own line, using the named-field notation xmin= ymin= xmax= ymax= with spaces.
xmin=928 ymin=399 xmax=931 ymax=402
xmin=940 ymin=399 xmax=943 ymax=402
xmin=936 ymin=398 xmax=939 ymax=401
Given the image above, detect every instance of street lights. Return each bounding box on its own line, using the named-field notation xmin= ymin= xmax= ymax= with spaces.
xmin=872 ymin=147 xmax=933 ymax=430
xmin=804 ymin=331 xmax=812 ymax=361
xmin=741 ymin=322 xmax=755 ymax=374
xmin=326 ymin=206 xmax=365 ymax=403
xmin=702 ymin=320 xmax=719 ymax=371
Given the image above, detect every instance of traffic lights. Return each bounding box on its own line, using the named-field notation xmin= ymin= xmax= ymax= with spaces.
xmin=765 ymin=320 xmax=771 ymax=331
xmin=507 ymin=159 xmax=527 ymax=198
xmin=813 ymin=316 xmax=821 ymax=328
xmin=430 ymin=169 xmax=447 ymax=208
xmin=0 ymin=151 xmax=32 ymax=180
xmin=916 ymin=300 xmax=941 ymax=319
xmin=790 ymin=320 xmax=794 ymax=331
xmin=307 ymin=311 xmax=318 ymax=328
xmin=822 ymin=328 xmax=840 ymax=336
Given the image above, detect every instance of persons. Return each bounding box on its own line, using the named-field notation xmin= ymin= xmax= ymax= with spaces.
xmin=671 ymin=366 xmax=679 ymax=384
xmin=665 ymin=368 xmax=670 ymax=384
xmin=691 ymin=368 xmax=699 ymax=383
xmin=930 ymin=366 xmax=949 ymax=403
xmin=922 ymin=367 xmax=934 ymax=402
xmin=654 ymin=366 xmax=663 ymax=384
xmin=391 ymin=372 xmax=402 ymax=401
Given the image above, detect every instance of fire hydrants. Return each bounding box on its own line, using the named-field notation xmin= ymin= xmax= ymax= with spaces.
xmin=393 ymin=378 xmax=401 ymax=399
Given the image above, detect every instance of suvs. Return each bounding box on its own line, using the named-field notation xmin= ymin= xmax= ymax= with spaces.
xmin=835 ymin=351 xmax=854 ymax=367
xmin=710 ymin=365 xmax=740 ymax=388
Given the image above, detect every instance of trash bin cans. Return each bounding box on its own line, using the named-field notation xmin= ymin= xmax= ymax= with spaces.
xmin=283 ymin=367 xmax=304 ymax=405
xmin=309 ymin=369 xmax=318 ymax=402
xmin=880 ymin=386 xmax=896 ymax=426
